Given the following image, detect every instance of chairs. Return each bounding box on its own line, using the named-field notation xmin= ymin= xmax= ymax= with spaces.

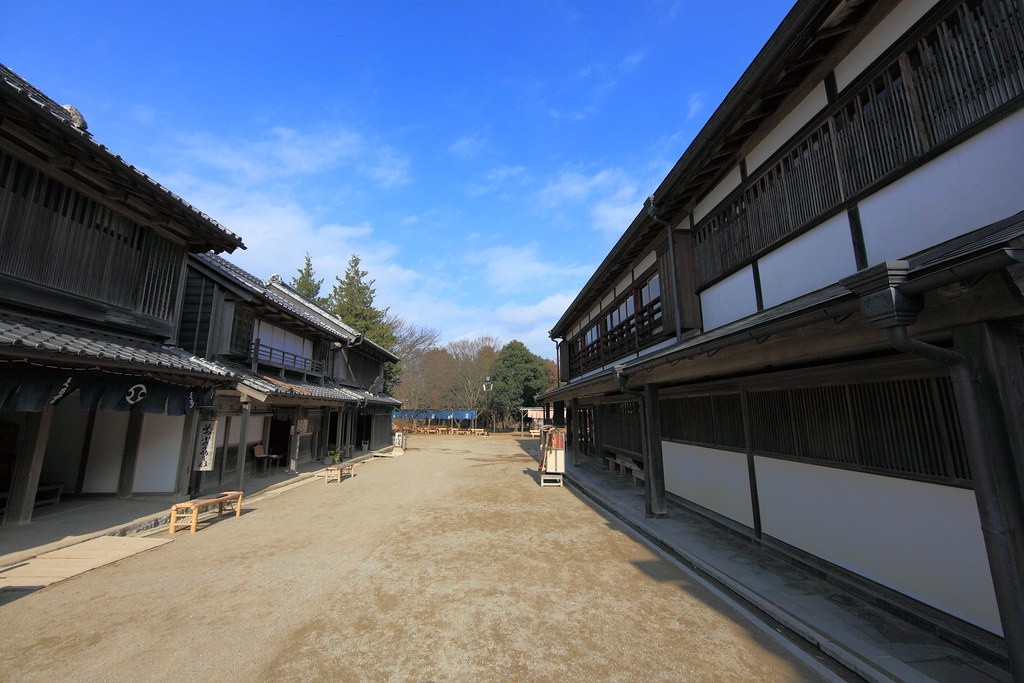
xmin=253 ymin=445 xmax=283 ymax=478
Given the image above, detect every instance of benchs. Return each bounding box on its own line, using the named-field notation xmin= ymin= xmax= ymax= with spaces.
xmin=325 ymin=463 xmax=354 ymax=485
xmin=168 ymin=490 xmax=244 ymax=536
